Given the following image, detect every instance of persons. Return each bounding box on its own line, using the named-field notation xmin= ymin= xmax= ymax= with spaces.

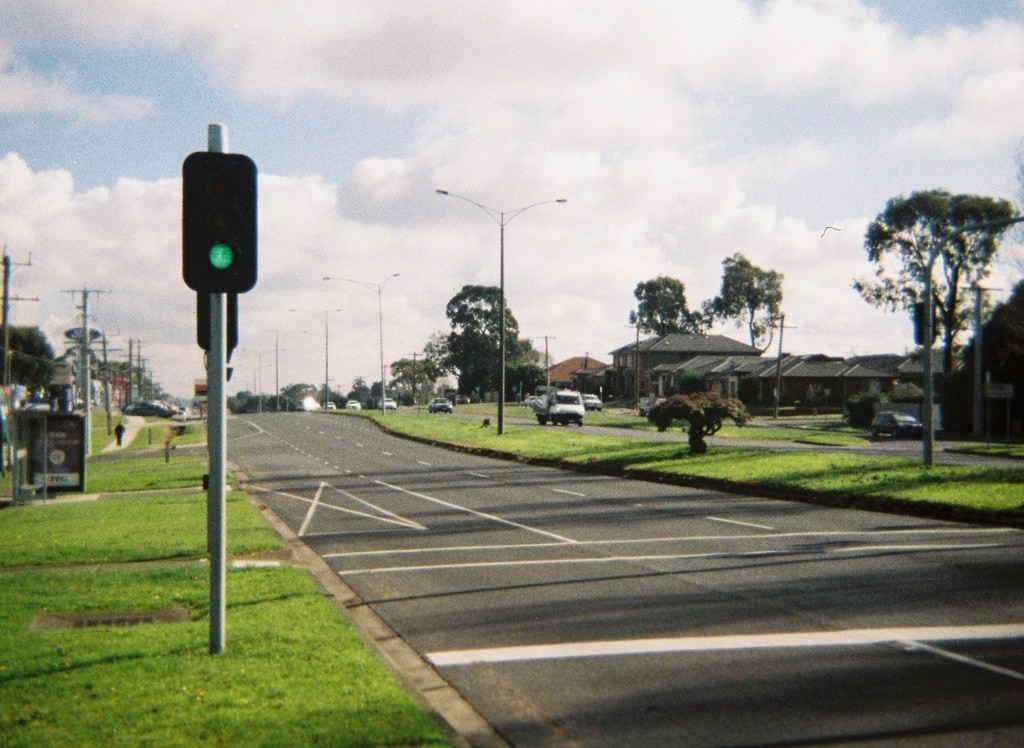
xmin=114 ymin=422 xmax=125 ymax=445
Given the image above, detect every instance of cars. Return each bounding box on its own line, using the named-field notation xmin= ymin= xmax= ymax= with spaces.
xmin=348 ymin=400 xmax=361 ymax=411
xmin=582 ymin=394 xmax=602 ymax=411
xmin=324 ymin=402 xmax=336 ymax=410
xmin=120 ymin=398 xmax=186 ymax=418
xmin=528 ymin=396 xmax=537 ymax=408
xmin=378 ymin=397 xmax=397 ymax=410
xmin=870 ymin=411 xmax=923 ymax=439
xmin=457 ymin=395 xmax=471 ymax=404
xmin=428 ymin=398 xmax=453 ymax=414
xmin=639 ymin=396 xmax=667 ymax=417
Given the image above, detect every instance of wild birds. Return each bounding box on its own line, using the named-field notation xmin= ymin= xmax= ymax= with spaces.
xmin=821 ymin=226 xmax=843 ymax=238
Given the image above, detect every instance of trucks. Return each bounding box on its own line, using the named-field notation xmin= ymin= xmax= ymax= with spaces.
xmin=533 ymin=390 xmax=584 ymax=427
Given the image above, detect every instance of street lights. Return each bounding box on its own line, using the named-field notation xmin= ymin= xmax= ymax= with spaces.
xmin=322 ymin=274 xmax=400 ymax=413
xmin=435 ymin=189 xmax=566 ymax=434
xmin=289 ymin=308 xmax=343 ymax=410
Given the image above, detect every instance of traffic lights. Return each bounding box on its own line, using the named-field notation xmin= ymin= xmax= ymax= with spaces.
xmin=182 ymin=152 xmax=259 ymax=293
